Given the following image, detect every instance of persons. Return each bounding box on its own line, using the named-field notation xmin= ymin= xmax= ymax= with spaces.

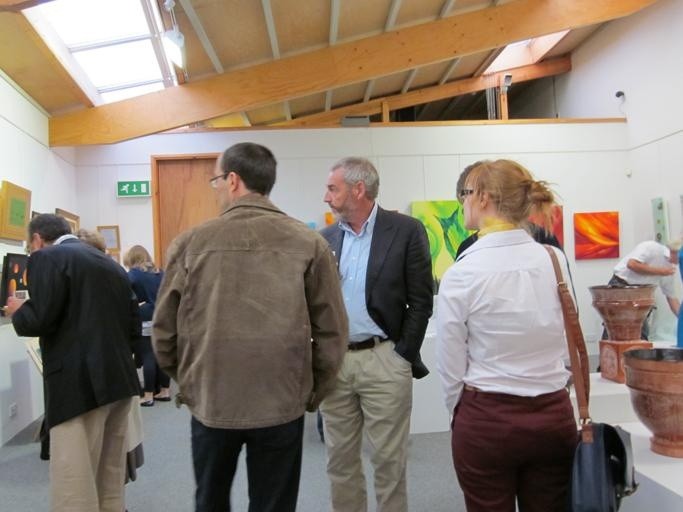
xmin=122 ymin=243 xmax=172 ymax=407
xmin=150 ymin=141 xmax=352 ymax=512
xmin=30 ymin=227 xmax=144 ymax=488
xmin=435 ymin=156 xmax=581 ymax=512
xmin=454 ymin=158 xmax=564 ymax=261
xmin=316 ymin=153 xmax=436 ymax=512
xmin=4 ymin=211 xmax=145 ymax=511
xmin=595 ymin=238 xmax=683 ymax=373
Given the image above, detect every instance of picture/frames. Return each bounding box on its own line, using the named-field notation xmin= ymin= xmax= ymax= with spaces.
xmin=31 ymin=211 xmax=38 ymax=219
xmin=96 ymin=225 xmax=120 ymax=252
xmin=0 ymin=252 xmax=29 ymax=317
xmin=55 ymin=208 xmax=81 ymax=233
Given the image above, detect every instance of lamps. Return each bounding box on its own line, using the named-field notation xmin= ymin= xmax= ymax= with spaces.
xmin=161 ymin=1 xmax=186 ymax=68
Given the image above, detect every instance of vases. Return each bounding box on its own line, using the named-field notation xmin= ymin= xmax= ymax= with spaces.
xmin=589 ymin=283 xmax=657 ymax=342
xmin=623 ymin=348 xmax=683 ymax=458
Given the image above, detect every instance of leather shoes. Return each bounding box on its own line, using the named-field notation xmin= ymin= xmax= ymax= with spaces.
xmin=140 ymin=401 xmax=154 ymax=406
xmin=153 ymin=395 xmax=170 ymax=401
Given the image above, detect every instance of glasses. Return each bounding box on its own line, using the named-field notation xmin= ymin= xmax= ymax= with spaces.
xmin=459 ymin=190 xmax=473 ymax=196
xmin=209 ymin=173 xmax=232 ymax=189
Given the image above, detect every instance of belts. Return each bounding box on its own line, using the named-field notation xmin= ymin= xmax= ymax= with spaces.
xmin=348 ymin=335 xmax=390 ymax=349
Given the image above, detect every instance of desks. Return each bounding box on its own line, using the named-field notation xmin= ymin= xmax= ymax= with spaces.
xmin=570 ymin=373 xmax=636 ymax=429
xmin=610 ymin=423 xmax=683 ymax=512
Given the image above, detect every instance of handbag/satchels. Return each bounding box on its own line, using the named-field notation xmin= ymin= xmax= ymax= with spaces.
xmin=572 ymin=422 xmax=640 ymax=512
